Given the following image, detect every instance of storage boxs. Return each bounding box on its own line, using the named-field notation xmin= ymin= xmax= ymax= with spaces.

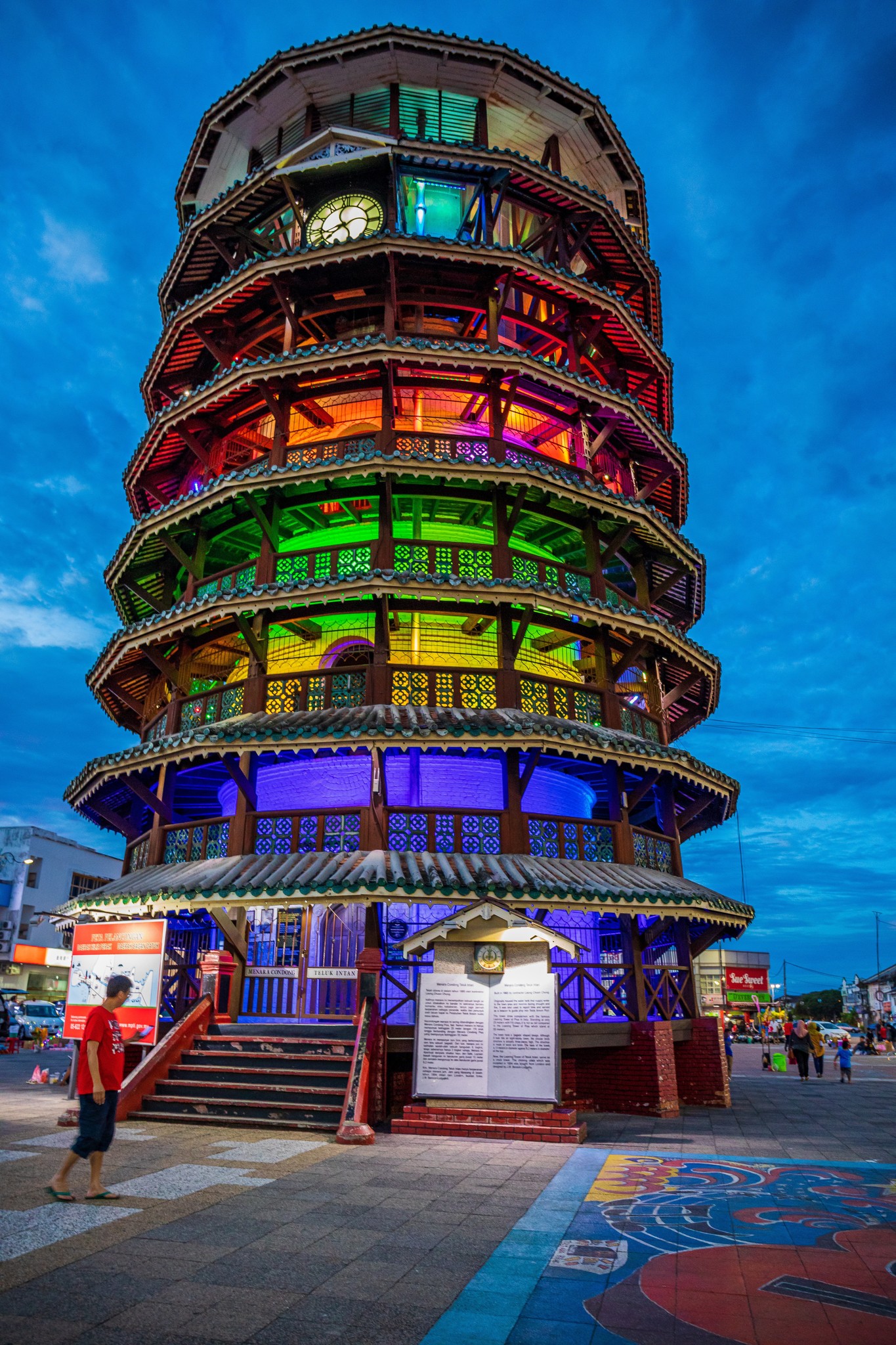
xmin=24 ymin=1040 xmax=35 ymax=1049
xmin=49 ymin=1036 xmax=69 ymax=1048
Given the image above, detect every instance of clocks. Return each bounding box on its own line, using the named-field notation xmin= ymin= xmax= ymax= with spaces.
xmin=299 ymin=183 xmax=388 ymax=249
xmin=474 ymin=942 xmax=506 ymax=974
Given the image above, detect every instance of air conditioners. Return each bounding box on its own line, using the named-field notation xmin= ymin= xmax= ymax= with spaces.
xmin=0 ymin=920 xmax=14 ymax=952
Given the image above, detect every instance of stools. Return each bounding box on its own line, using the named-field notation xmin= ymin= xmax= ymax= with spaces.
xmin=5 ymin=1036 xmax=19 ymax=1054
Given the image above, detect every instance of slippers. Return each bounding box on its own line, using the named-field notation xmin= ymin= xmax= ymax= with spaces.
xmin=44 ymin=1186 xmax=76 ymax=1202
xmin=84 ymin=1191 xmax=119 ymax=1200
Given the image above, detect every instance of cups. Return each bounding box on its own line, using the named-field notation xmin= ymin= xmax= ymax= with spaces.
xmin=55 ymin=1073 xmax=60 ymax=1080
xmin=49 ymin=1074 xmax=55 ymax=1084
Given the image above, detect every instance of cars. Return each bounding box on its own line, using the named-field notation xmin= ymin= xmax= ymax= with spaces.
xmin=836 ymin=1023 xmax=862 ymax=1033
xmin=805 ymin=1021 xmax=850 ymax=1044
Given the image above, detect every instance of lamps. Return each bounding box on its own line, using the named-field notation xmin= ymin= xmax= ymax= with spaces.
xmin=77 ymin=908 xmax=154 ymax=924
xmin=29 ymin=911 xmax=77 ymax=926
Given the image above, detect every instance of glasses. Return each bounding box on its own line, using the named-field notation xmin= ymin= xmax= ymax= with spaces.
xmin=120 ymin=990 xmax=132 ymax=998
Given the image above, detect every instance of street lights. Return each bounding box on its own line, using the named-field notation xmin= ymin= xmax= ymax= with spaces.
xmin=770 ymin=984 xmax=780 ymax=1003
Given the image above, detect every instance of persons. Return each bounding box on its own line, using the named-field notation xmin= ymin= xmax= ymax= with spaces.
xmin=723 ymin=1028 xmax=738 ymax=1080
xmin=784 ymin=1017 xmax=794 ymax=1051
xmin=833 ymin=1040 xmax=854 ymax=1083
xmin=791 ymin=1019 xmax=815 ymax=1080
xmin=852 ymin=1019 xmax=891 ymax=1055
xmin=46 ymin=975 xmax=141 ymax=1200
xmin=9 ymin=995 xmax=25 ymax=1018
xmin=769 ymin=1017 xmax=780 ymax=1044
xmin=804 ymin=1022 xmax=826 ymax=1078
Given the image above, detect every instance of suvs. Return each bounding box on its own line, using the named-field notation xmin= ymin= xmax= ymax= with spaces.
xmin=0 ymin=988 xmax=67 ymax=1042
